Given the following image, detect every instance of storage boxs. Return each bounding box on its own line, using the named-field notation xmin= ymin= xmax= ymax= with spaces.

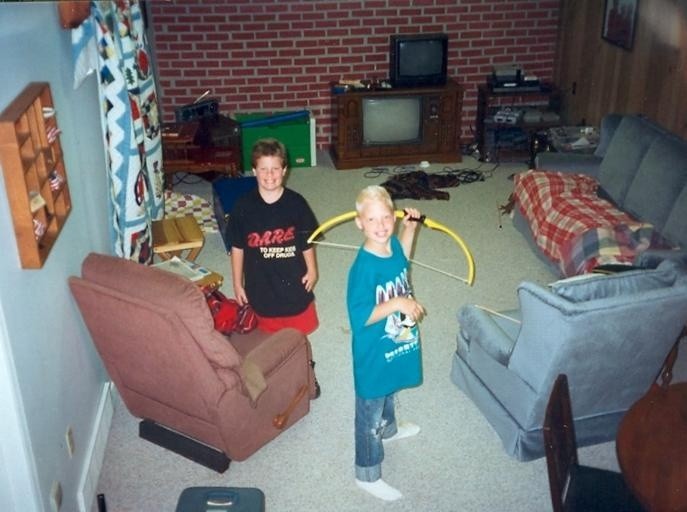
xmin=175 ymin=486 xmax=264 ymax=512
xmin=232 ymin=111 xmax=317 ymax=174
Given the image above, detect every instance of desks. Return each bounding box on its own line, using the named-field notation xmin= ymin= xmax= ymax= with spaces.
xmin=163 ymin=114 xmax=241 ymax=193
xmin=329 ymin=79 xmax=465 ymax=170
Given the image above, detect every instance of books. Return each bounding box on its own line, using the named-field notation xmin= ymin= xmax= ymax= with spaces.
xmin=151 ymin=256 xmax=211 ymax=284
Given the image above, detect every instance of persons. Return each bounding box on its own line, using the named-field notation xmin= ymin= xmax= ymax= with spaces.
xmin=227 ymin=138 xmax=323 ymax=401
xmin=346 ymin=184 xmax=426 ymax=501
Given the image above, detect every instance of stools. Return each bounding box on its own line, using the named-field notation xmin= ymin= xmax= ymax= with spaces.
xmin=195 ymin=265 xmax=223 ymax=289
xmin=152 ymin=216 xmax=204 ymax=262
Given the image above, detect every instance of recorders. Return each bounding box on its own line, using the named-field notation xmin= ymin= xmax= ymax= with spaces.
xmin=175 ymin=100 xmax=218 ymax=124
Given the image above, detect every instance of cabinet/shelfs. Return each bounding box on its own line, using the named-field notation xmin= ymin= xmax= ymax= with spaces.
xmin=0 ymin=81 xmax=72 ymax=270
xmin=476 ymin=84 xmax=562 ymax=159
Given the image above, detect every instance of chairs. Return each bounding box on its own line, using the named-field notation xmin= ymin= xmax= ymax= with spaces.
xmin=69 ymin=279 xmax=316 ymax=462
xmin=449 ymin=281 xmax=686 ymax=463
xmin=544 ymin=375 xmax=644 ymax=512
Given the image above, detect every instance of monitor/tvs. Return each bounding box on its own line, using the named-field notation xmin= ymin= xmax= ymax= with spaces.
xmin=362 ymin=96 xmax=423 ymax=145
xmin=389 ymin=34 xmax=448 ymax=88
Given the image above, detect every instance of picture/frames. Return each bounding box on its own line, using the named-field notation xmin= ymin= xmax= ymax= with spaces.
xmin=601 ymin=0 xmax=638 ymax=50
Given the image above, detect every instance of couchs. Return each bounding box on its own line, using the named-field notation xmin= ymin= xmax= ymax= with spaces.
xmin=514 ymin=112 xmax=687 ymax=279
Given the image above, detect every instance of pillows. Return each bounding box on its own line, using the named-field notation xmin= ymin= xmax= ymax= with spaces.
xmin=550 ymin=260 xmax=679 ymax=300
xmin=81 ymin=253 xmax=242 ymax=370
